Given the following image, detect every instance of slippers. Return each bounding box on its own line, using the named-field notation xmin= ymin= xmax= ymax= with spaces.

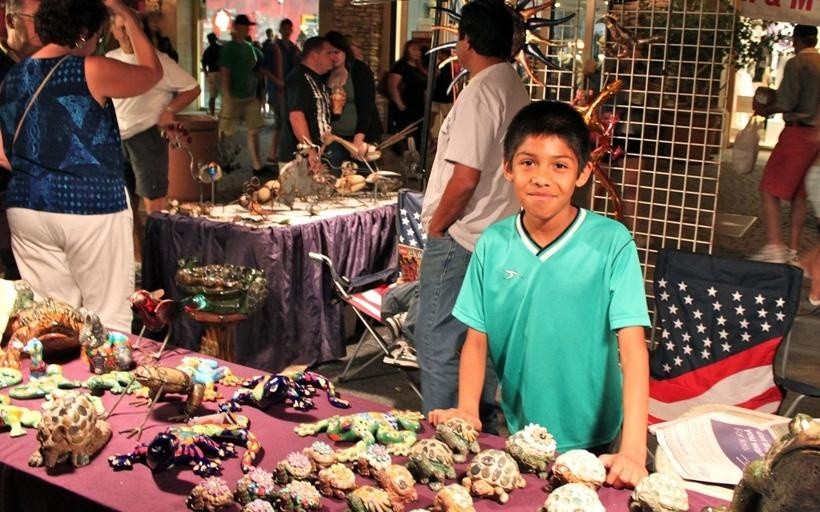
xmin=789 ymin=260 xmax=812 ymax=281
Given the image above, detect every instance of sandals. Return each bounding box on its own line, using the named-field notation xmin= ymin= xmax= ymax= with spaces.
xmin=430 ymin=138 xmax=437 ymax=154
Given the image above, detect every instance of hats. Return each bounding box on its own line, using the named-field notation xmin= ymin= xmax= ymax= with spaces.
xmin=785 ymin=25 xmax=817 ymax=39
xmin=233 ymin=14 xmax=257 ymax=25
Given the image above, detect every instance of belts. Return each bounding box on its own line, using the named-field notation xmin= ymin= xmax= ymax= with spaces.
xmin=786 ymin=121 xmax=814 ymax=127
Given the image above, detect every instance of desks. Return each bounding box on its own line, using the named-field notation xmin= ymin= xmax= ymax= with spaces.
xmin=136 ymin=181 xmax=412 ymax=376
xmin=1 ymin=316 xmax=736 ymax=512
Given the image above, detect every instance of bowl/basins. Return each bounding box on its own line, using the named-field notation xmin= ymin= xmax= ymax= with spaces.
xmin=174 ymin=258 xmax=269 ymax=316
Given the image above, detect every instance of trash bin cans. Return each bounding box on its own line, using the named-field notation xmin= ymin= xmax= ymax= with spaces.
xmin=162 ymin=114 xmax=219 ymax=202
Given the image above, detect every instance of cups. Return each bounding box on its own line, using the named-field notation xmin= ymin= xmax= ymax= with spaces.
xmin=330 ymin=86 xmax=347 ymax=116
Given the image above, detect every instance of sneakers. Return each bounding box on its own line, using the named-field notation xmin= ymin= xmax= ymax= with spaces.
xmin=381 ymin=338 xmax=420 ymax=369
xmin=385 ymin=310 xmax=407 ymax=337
xmin=784 ymin=246 xmax=800 ymax=265
xmin=253 ymin=167 xmax=278 ymax=180
xmin=747 ymin=244 xmax=787 ymax=264
xmin=265 ymin=156 xmax=277 ymax=166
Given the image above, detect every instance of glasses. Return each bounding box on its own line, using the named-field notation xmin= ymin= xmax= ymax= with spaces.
xmin=94 ymin=30 xmax=104 ymax=47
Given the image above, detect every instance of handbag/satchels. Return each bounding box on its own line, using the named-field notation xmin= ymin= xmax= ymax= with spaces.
xmin=376 ymin=72 xmax=405 ymax=101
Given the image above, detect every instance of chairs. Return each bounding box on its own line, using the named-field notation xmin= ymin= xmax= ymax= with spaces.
xmin=305 ymin=187 xmax=434 ymax=404
xmin=603 ymin=245 xmax=819 ymax=501
